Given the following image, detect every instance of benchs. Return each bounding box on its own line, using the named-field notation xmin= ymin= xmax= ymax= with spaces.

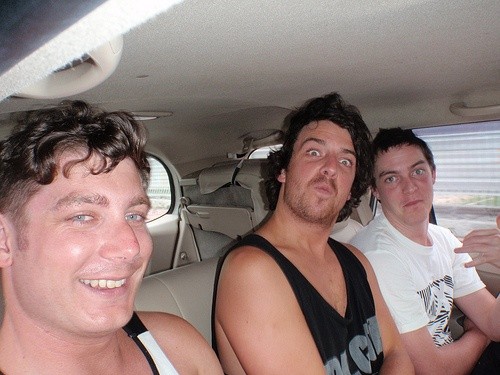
xmin=133 ymin=220 xmax=363 ymax=350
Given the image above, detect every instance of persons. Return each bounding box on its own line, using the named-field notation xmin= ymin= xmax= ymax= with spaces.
xmin=351 ymin=127 xmax=500 ymax=375
xmin=214 ymin=92 xmax=415 ymax=375
xmin=0 ymin=99 xmax=225 ymax=375
